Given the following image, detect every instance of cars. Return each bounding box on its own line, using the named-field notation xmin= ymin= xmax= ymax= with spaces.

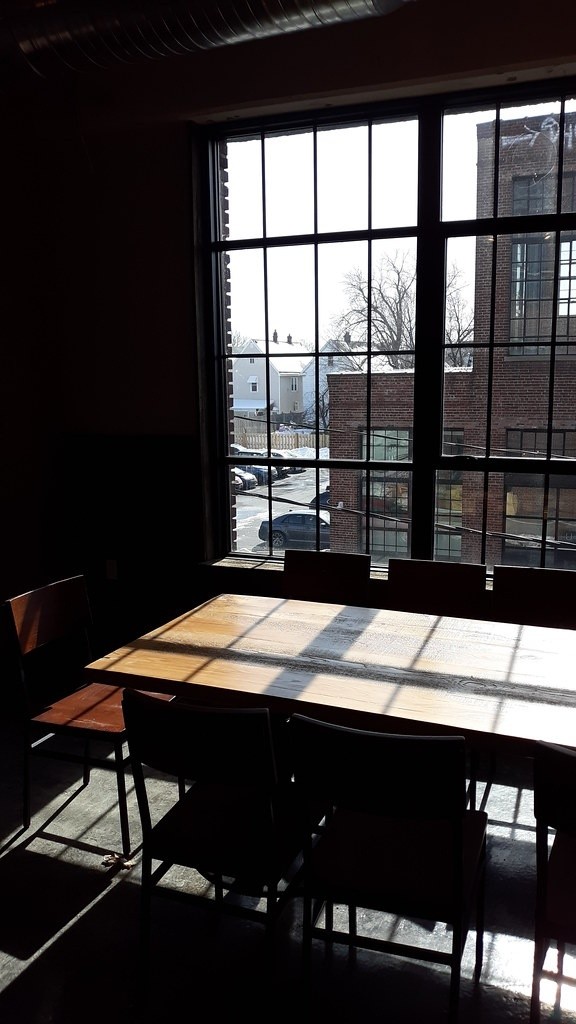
xmin=229 ymin=444 xmax=307 ymax=491
xmin=258 ymin=509 xmax=331 ymax=547
xmin=307 ymin=491 xmax=331 ymax=510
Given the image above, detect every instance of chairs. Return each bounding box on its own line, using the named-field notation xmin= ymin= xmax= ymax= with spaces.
xmin=6 ymin=551 xmax=576 ymax=1011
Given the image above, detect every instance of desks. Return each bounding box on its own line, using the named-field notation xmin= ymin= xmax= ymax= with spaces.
xmin=86 ymin=593 xmax=576 ymax=870
